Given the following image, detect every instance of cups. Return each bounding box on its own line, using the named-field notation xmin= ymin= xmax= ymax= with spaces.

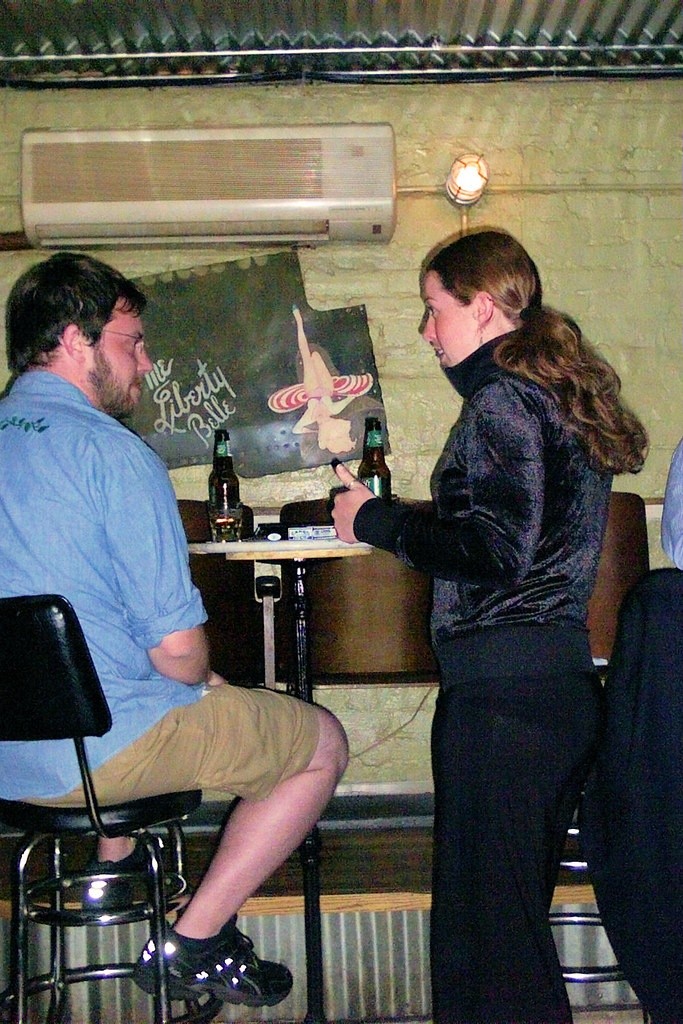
xmin=209 ymin=507 xmax=243 ymax=542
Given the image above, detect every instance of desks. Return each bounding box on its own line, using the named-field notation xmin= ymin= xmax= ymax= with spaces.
xmin=192 ymin=537 xmax=376 ymax=1024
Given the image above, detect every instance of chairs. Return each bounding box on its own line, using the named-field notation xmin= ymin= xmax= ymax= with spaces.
xmin=0 ymin=594 xmax=223 ymax=1024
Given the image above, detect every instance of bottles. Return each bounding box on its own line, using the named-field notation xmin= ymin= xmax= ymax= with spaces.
xmin=359 ymin=422 xmax=392 ymax=501
xmin=208 ymin=431 xmax=239 ymax=508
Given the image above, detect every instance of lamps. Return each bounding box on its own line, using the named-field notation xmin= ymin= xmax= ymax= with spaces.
xmin=447 ymin=153 xmax=489 ymax=204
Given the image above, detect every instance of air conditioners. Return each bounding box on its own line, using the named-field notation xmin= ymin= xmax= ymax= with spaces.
xmin=20 ymin=122 xmax=398 ymax=246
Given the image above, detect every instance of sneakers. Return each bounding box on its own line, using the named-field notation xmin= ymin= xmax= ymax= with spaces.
xmin=131 ymin=907 xmax=294 ymax=1006
xmin=80 ymin=834 xmax=186 ymax=912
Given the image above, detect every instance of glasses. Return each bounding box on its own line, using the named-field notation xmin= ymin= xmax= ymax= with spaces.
xmin=72 ymin=321 xmax=144 ymax=352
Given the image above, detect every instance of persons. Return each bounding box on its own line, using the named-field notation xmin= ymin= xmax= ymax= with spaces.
xmin=329 ymin=231 xmax=651 ymax=1024
xmin=0 ymin=249 xmax=350 ymax=1010
